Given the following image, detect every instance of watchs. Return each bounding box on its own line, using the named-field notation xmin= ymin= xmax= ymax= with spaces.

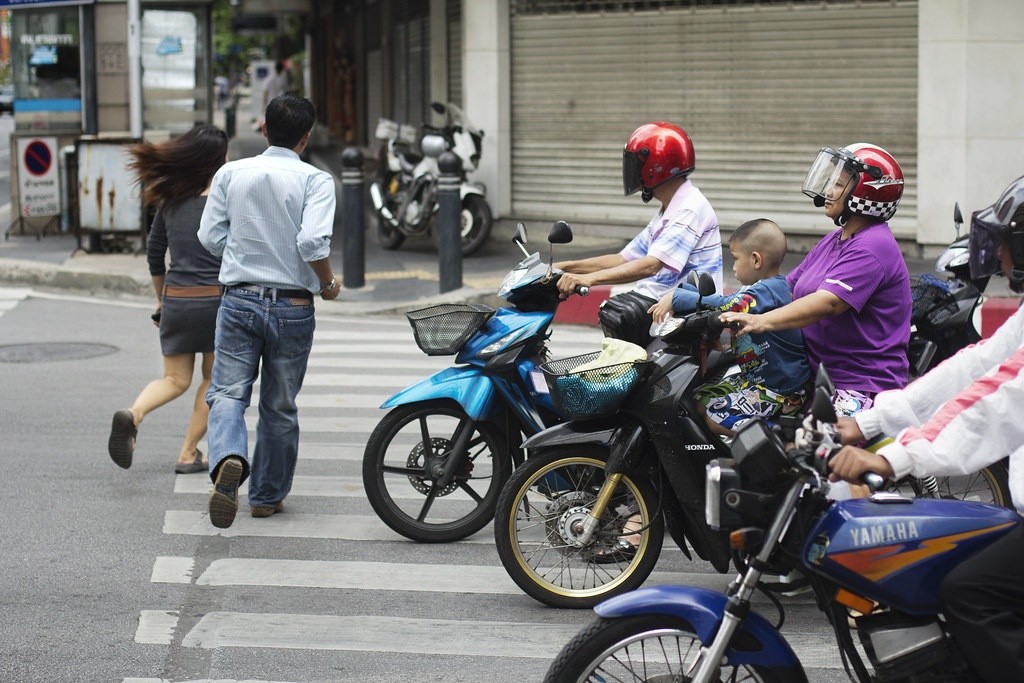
xmin=319 ymin=272 xmax=336 ymax=290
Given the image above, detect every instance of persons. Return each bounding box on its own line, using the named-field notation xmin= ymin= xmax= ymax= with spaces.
xmin=648 ymin=217 xmax=812 ymax=450
xmin=555 ymin=121 xmax=724 ymax=361
xmin=197 ymin=95 xmax=341 ymax=529
xmin=719 ymin=143 xmax=912 ymax=446
xmin=107 ymin=124 xmax=228 ymax=472
xmin=827 ymin=173 xmax=1024 ymax=683
xmin=262 ymin=63 xmax=287 ymax=104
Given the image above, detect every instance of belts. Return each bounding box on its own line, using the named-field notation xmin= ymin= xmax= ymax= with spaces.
xmin=161 ymin=285 xmax=222 ymax=298
xmin=238 ymin=281 xmax=311 ymax=298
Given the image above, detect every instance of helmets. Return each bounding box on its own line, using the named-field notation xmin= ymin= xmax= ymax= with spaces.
xmin=968 ymin=174 xmax=1024 ymax=292
xmin=622 ymin=120 xmax=694 ymax=197
xmin=830 ymin=143 xmax=905 ymax=222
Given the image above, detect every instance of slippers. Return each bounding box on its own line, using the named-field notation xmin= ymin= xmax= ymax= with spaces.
xmin=174 ymin=447 xmax=208 ymax=474
xmin=108 ymin=409 xmax=137 ymax=470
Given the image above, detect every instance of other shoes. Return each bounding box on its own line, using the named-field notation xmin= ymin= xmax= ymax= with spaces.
xmin=209 ymin=458 xmax=243 ymax=529
xmin=249 ymin=503 xmax=283 ymax=517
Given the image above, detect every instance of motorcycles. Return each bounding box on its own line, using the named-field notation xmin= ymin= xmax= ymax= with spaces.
xmin=362 ymin=224 xmax=1016 ymax=609
xmin=371 ymin=99 xmax=491 ymax=256
xmin=909 ymin=202 xmax=996 ymax=376
xmin=543 ymin=358 xmax=1018 ymax=683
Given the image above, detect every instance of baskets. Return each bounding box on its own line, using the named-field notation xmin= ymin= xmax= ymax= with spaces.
xmin=404 ymin=303 xmax=496 ymax=358
xmin=539 ymin=349 xmax=654 ymax=421
